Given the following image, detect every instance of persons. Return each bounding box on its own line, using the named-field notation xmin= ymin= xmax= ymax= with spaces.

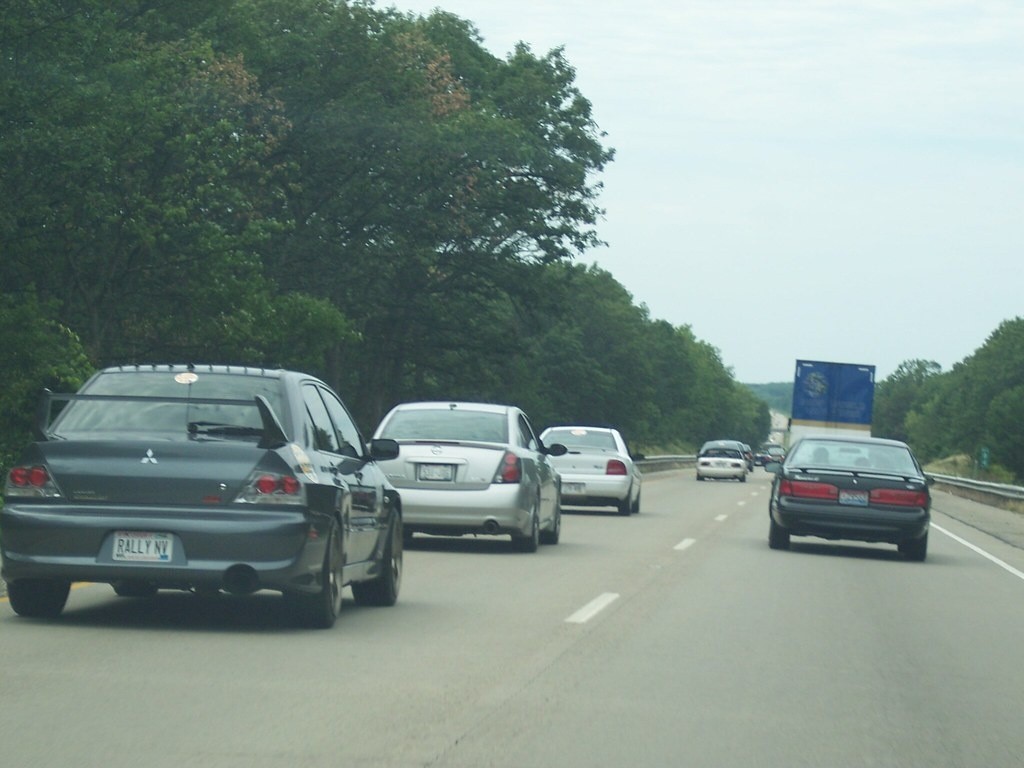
xmin=811 ymin=447 xmax=830 ymax=465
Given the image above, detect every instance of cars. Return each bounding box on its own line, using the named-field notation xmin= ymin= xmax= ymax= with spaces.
xmin=768 ymin=435 xmax=936 ymax=560
xmin=694 ymin=440 xmax=754 ymax=482
xmin=539 ymin=426 xmax=642 ymax=515
xmin=0 ymin=366 xmax=403 ymax=630
xmin=755 ymin=443 xmax=786 ymax=467
xmin=370 ymin=402 xmax=563 ymax=552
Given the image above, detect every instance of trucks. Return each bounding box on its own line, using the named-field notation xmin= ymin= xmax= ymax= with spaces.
xmin=786 ymin=358 xmax=875 ymax=453
